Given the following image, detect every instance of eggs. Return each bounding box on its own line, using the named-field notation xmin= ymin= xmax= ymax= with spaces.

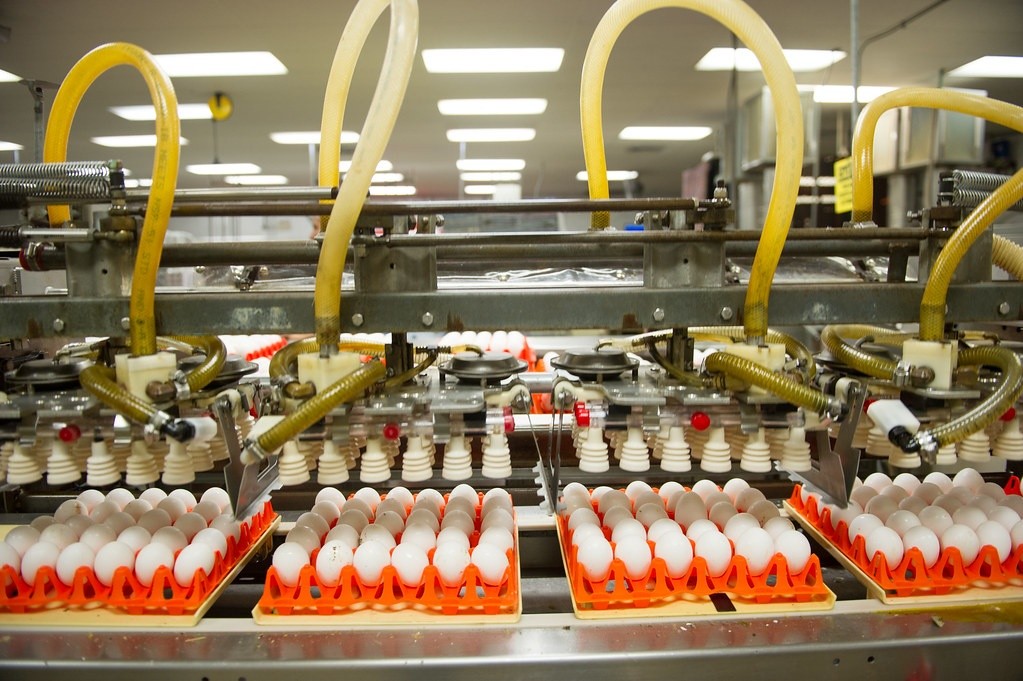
xmin=0 ymin=469 xmax=1022 ymax=586
xmin=218 ymin=335 xmax=281 ymax=356
xmin=440 ymin=331 xmax=525 ymax=356
